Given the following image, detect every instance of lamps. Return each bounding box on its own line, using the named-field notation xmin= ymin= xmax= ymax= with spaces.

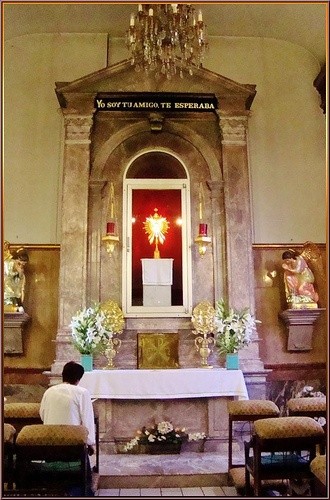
xmin=125 ymin=4 xmax=207 ymax=80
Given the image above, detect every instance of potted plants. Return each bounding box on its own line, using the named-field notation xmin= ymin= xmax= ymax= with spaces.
xmin=214 ymin=297 xmax=262 ymax=371
xmin=70 ymin=300 xmax=113 ymax=373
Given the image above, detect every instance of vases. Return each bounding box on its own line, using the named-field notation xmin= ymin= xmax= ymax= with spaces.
xmin=145 ymin=440 xmax=182 ymax=454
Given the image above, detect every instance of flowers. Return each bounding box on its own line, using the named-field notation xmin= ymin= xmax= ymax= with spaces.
xmin=118 ymin=418 xmax=206 ymax=453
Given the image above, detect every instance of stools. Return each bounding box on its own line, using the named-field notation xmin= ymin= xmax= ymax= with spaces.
xmin=226 ymin=397 xmax=326 ymax=496
xmin=3 ymin=383 xmax=89 ymax=497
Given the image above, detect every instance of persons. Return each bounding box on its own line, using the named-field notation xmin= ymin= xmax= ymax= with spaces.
xmin=282 ymin=247 xmax=318 ymax=302
xmin=3 ymin=241 xmax=28 ymax=305
xmin=39 ymin=362 xmax=95 ymax=456
